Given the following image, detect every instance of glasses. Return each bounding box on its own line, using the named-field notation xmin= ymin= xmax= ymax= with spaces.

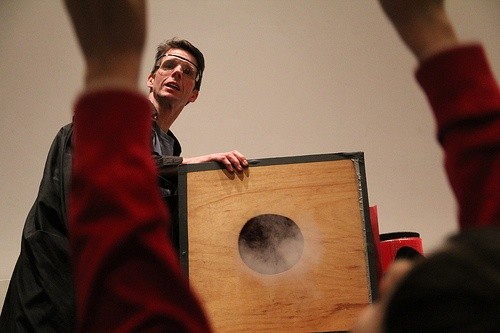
xmin=154 ymin=54 xmax=200 ymax=82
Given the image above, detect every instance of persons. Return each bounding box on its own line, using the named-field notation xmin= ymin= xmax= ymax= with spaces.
xmin=58 ymin=0 xmax=499 ymax=333
xmin=1 ymin=36 xmax=248 ymax=333
